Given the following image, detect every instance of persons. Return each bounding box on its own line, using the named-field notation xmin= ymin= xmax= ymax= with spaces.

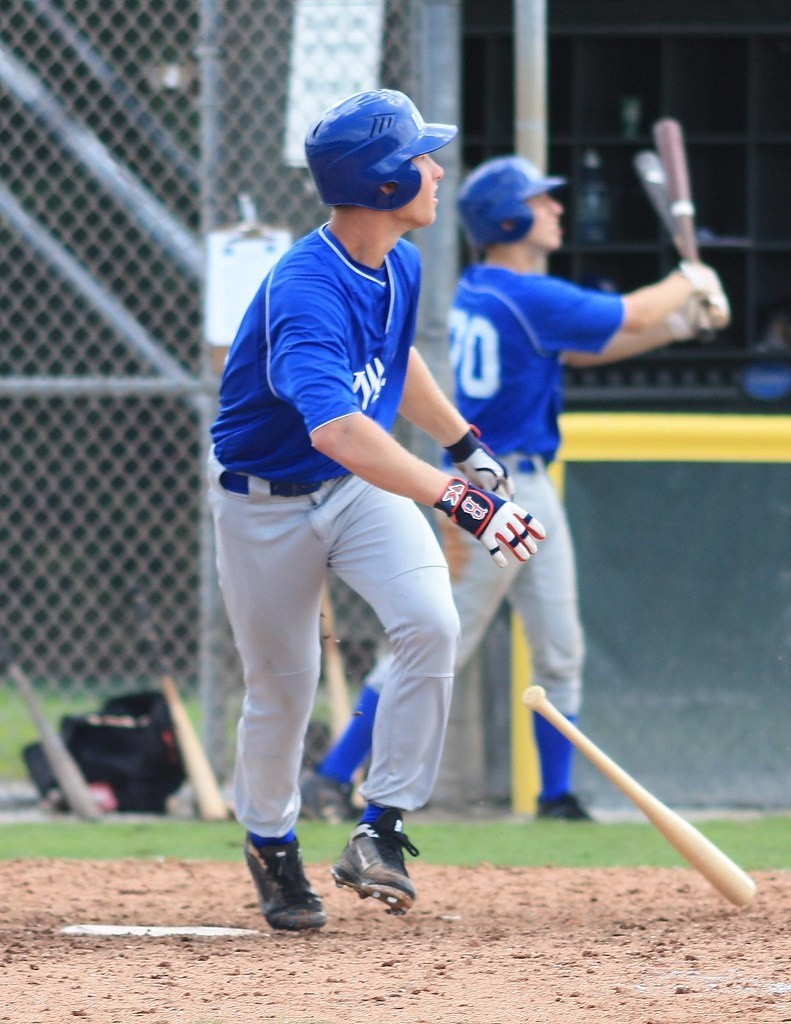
xmin=301 ymin=156 xmax=731 ymax=822
xmin=207 ymin=91 xmax=550 ymax=932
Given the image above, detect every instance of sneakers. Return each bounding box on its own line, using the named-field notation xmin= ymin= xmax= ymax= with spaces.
xmin=295 ymin=772 xmax=363 ymax=822
xmin=330 ymin=807 xmax=419 ymax=913
xmin=245 ymin=829 xmax=327 ymax=930
xmin=538 ymin=791 xmax=592 ymax=822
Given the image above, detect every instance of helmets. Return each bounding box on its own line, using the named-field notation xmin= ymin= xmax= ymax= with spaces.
xmin=455 ymin=154 xmax=568 ymax=249
xmin=303 ymin=88 xmax=457 ymax=212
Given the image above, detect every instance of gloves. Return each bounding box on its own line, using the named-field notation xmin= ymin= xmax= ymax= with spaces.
xmin=434 ymin=477 xmax=547 ymax=568
xmin=669 ymin=264 xmax=730 ymax=340
xmin=444 ymin=423 xmax=515 ymax=503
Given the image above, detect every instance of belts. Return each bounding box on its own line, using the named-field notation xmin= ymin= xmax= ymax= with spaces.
xmin=219 ymin=469 xmax=323 ymax=496
xmin=516 ymin=459 xmax=535 ymax=474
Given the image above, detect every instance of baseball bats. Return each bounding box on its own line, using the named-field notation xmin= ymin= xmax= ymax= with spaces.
xmin=126 ymin=584 xmax=230 ymax=821
xmin=632 ymin=113 xmax=719 ymax=348
xmin=0 ymin=635 xmax=108 ymax=824
xmin=521 ymin=681 xmax=760 ymax=910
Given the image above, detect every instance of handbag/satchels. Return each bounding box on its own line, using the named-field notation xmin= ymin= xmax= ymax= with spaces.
xmin=24 ymin=688 xmax=183 ymax=817
